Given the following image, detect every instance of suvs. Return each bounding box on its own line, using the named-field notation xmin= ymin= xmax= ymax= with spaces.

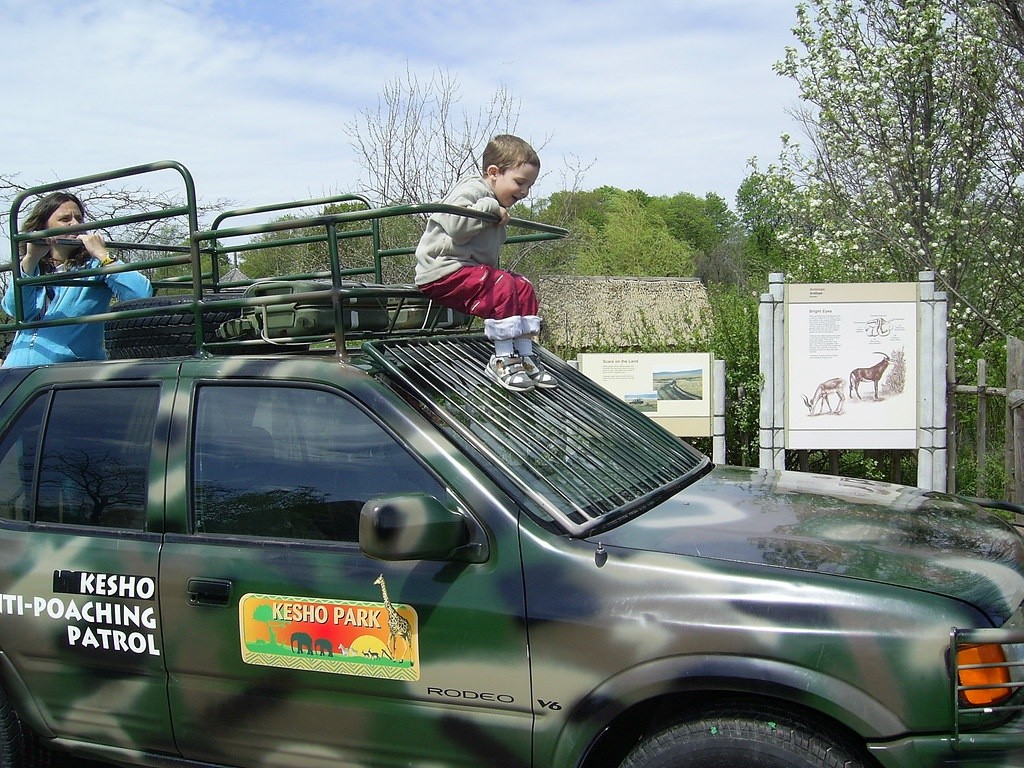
xmin=0 ymin=158 xmax=1023 ymax=768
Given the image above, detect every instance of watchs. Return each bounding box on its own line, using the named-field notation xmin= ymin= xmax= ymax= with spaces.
xmin=98 ymin=252 xmax=118 ymax=267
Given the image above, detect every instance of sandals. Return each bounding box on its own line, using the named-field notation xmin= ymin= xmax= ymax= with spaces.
xmin=485 ymin=353 xmax=534 ymax=391
xmin=519 ymin=354 xmax=558 ymax=388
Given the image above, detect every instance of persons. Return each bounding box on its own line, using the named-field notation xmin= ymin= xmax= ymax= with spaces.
xmin=414 ymin=136 xmax=560 ymax=392
xmin=2 ymin=192 xmax=153 ymax=368
